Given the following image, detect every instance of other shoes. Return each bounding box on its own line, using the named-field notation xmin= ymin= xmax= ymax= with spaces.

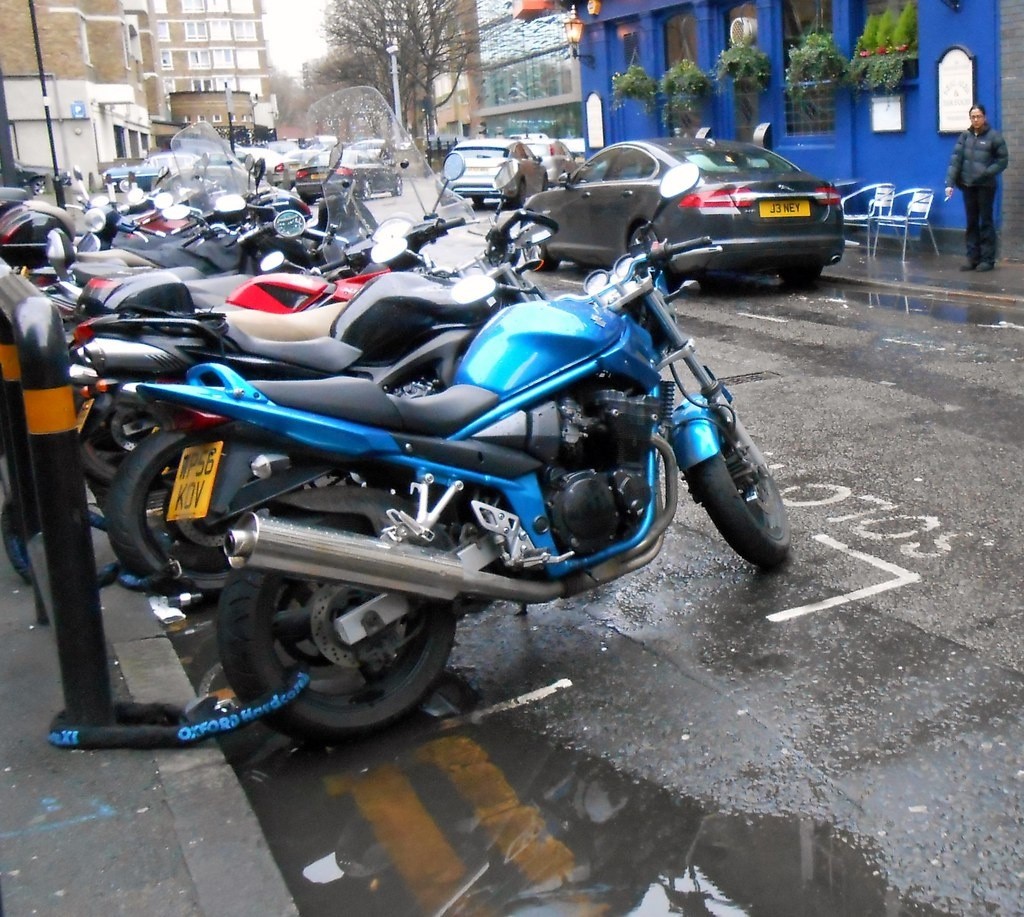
xmin=958 ymin=259 xmax=973 ymax=272
xmin=975 ymin=260 xmax=994 ymax=272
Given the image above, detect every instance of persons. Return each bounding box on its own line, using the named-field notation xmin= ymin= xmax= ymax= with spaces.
xmin=945 ymin=104 xmax=1008 ymax=271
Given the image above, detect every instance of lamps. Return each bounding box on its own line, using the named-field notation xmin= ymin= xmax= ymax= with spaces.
xmin=563 ymin=5 xmax=595 ymax=69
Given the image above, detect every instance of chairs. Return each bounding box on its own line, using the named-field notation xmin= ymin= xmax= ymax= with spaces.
xmin=872 ymin=187 xmax=940 ymax=262
xmin=838 ymin=183 xmax=895 ymax=256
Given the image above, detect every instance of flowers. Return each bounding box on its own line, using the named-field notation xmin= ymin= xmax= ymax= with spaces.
xmin=784 ymin=2 xmax=916 ymax=119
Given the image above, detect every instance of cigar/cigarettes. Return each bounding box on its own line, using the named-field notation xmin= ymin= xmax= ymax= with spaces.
xmin=945 ymin=197 xmax=949 ymax=202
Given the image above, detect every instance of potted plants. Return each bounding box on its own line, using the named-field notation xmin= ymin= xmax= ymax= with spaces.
xmin=660 ymin=60 xmax=709 ymax=137
xmin=712 ymin=46 xmax=771 ymax=96
xmin=611 ymin=66 xmax=657 ymax=116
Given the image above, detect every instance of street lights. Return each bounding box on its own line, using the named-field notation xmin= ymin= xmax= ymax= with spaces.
xmin=386 ymin=44 xmax=403 ymax=159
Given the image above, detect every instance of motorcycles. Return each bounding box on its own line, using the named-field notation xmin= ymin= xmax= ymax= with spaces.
xmin=0 ymin=85 xmax=797 ymax=749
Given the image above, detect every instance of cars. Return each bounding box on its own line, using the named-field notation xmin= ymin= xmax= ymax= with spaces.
xmin=297 ymin=150 xmax=403 ymax=207
xmin=1 ymin=162 xmax=72 ymax=197
xmin=515 ymin=136 xmax=580 ymax=189
xmin=269 ymin=134 xmax=382 ymax=155
xmin=218 ymin=148 xmax=305 ymax=186
xmin=149 ymin=164 xmax=313 ymax=235
xmin=435 ymin=136 xmax=550 ymax=211
xmin=519 ymin=136 xmax=848 ymax=287
xmin=104 ymin=150 xmax=210 ymax=193
